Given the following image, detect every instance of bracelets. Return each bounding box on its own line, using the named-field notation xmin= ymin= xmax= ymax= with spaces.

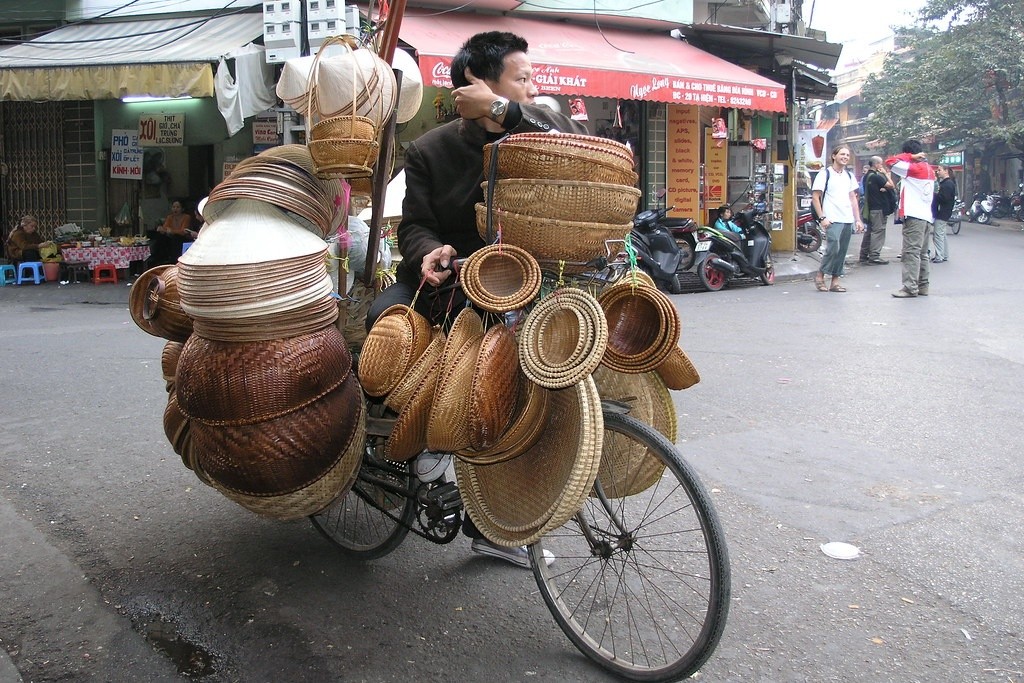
xmin=819 ymin=217 xmax=826 ymax=221
xmin=884 ymin=172 xmax=887 ymax=174
xmin=856 ymin=220 xmax=861 ymax=222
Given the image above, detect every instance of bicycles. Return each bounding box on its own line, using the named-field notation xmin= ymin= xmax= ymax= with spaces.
xmin=310 ymin=258 xmax=731 ymax=683
xmin=947 ymin=196 xmax=964 ymax=235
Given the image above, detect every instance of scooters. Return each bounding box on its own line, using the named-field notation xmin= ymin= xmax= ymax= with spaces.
xmin=629 ymin=187 xmax=682 ymax=295
xmin=986 ymin=183 xmax=1024 ymax=222
xmin=966 ymin=192 xmax=997 ymax=224
xmin=694 ymin=194 xmax=775 ymax=292
xmin=634 ymin=217 xmax=698 ymax=271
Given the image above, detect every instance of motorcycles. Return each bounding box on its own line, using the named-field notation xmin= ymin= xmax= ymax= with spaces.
xmin=797 ymin=209 xmax=822 ymax=253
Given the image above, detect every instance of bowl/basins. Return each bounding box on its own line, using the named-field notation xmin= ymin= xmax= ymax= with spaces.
xmin=94 ymin=237 xmax=103 ymax=241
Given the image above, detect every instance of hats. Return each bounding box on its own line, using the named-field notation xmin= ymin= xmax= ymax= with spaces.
xmin=177 ymin=43 xmax=423 ymax=342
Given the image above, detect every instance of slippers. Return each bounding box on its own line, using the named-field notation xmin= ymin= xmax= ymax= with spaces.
xmin=829 ymin=285 xmax=846 ymax=291
xmin=814 ymin=277 xmax=828 ymax=292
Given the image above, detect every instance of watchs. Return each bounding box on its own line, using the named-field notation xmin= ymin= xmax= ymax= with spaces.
xmin=490 ymin=97 xmax=508 ymax=122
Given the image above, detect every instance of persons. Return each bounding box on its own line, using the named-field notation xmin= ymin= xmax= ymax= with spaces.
xmin=713 ymin=206 xmax=750 ymax=241
xmin=134 ymin=197 xmax=205 ymax=278
xmin=365 ymin=31 xmax=591 ymax=569
xmin=7 ymin=216 xmax=50 ymax=278
xmin=885 ymin=141 xmax=936 ymax=298
xmin=859 ymin=155 xmax=956 ymax=266
xmin=812 ymin=145 xmax=864 ymax=291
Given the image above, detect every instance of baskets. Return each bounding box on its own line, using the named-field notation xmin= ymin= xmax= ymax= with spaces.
xmin=129 ymin=264 xmax=366 ymax=522
xmin=305 ymin=34 xmax=379 ymax=179
xmin=357 ymin=132 xmax=700 ymax=546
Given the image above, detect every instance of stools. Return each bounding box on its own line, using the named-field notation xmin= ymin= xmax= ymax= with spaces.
xmin=92 ymin=264 xmax=118 ymax=285
xmin=63 ymin=260 xmax=90 ymax=282
xmin=0 ymin=264 xmax=18 ymax=287
xmin=18 ymin=261 xmax=47 ymax=285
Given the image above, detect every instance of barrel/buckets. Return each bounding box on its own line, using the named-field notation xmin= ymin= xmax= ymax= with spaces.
xmin=44 ymin=261 xmax=59 ymax=280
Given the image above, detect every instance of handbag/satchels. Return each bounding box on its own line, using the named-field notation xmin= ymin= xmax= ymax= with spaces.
xmin=114 ymin=202 xmax=133 ymax=227
xmin=882 ymin=190 xmax=899 ymax=215
xmin=860 ymin=203 xmax=870 ymax=224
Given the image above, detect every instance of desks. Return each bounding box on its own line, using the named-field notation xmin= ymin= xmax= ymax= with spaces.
xmin=61 ymin=245 xmax=151 ymax=280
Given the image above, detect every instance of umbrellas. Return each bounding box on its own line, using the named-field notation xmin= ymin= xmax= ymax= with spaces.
xmin=114 ymin=202 xmax=133 ymax=235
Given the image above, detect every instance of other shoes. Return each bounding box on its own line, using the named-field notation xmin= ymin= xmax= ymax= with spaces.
xmin=891 ymin=290 xmax=918 ymax=297
xmin=918 ymin=290 xmax=928 ymax=296
xmin=897 ymin=254 xmax=902 ymax=258
xmin=860 ymin=256 xmax=869 ymax=261
xmin=933 ymin=257 xmax=943 ymax=262
xmin=931 ymin=256 xmax=947 ymax=261
xmin=868 ymin=259 xmax=889 ymax=264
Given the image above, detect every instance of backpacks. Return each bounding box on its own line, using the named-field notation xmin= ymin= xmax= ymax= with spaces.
xmin=811 ymin=168 xmax=851 ymax=226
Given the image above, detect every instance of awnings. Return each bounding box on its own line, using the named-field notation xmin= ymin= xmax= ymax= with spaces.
xmin=0 ymin=12 xmax=264 ymax=101
xmin=356 ymin=4 xmax=787 ymax=114
xmin=993 ymin=150 xmax=1024 ymax=160
xmin=817 ymin=119 xmax=839 ymax=133
xmin=779 ymin=33 xmax=844 ymax=101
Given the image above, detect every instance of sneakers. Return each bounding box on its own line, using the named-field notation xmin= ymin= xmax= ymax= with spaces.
xmin=416 ymin=449 xmax=451 ymax=483
xmin=472 ymin=535 xmax=555 ymax=569
